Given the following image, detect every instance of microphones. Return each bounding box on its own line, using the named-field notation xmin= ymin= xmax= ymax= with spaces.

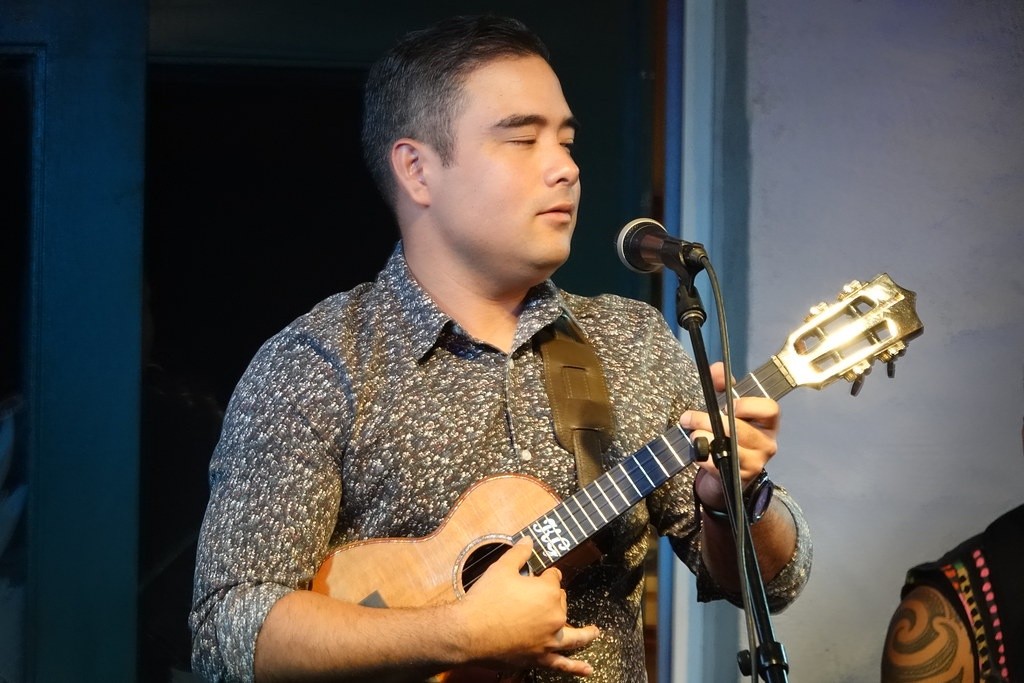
xmin=613 ymin=215 xmax=709 ymax=275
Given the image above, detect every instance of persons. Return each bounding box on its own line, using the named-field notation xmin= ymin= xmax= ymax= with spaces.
xmin=189 ymin=8 xmax=812 ymax=683
xmin=881 ymin=499 xmax=1023 ymax=683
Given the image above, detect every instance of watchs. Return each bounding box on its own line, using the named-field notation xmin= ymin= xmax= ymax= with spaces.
xmin=691 ymin=458 xmax=774 ymax=527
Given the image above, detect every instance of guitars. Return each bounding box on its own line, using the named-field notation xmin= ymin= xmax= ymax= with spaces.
xmin=311 ymin=270 xmax=930 ymax=683
